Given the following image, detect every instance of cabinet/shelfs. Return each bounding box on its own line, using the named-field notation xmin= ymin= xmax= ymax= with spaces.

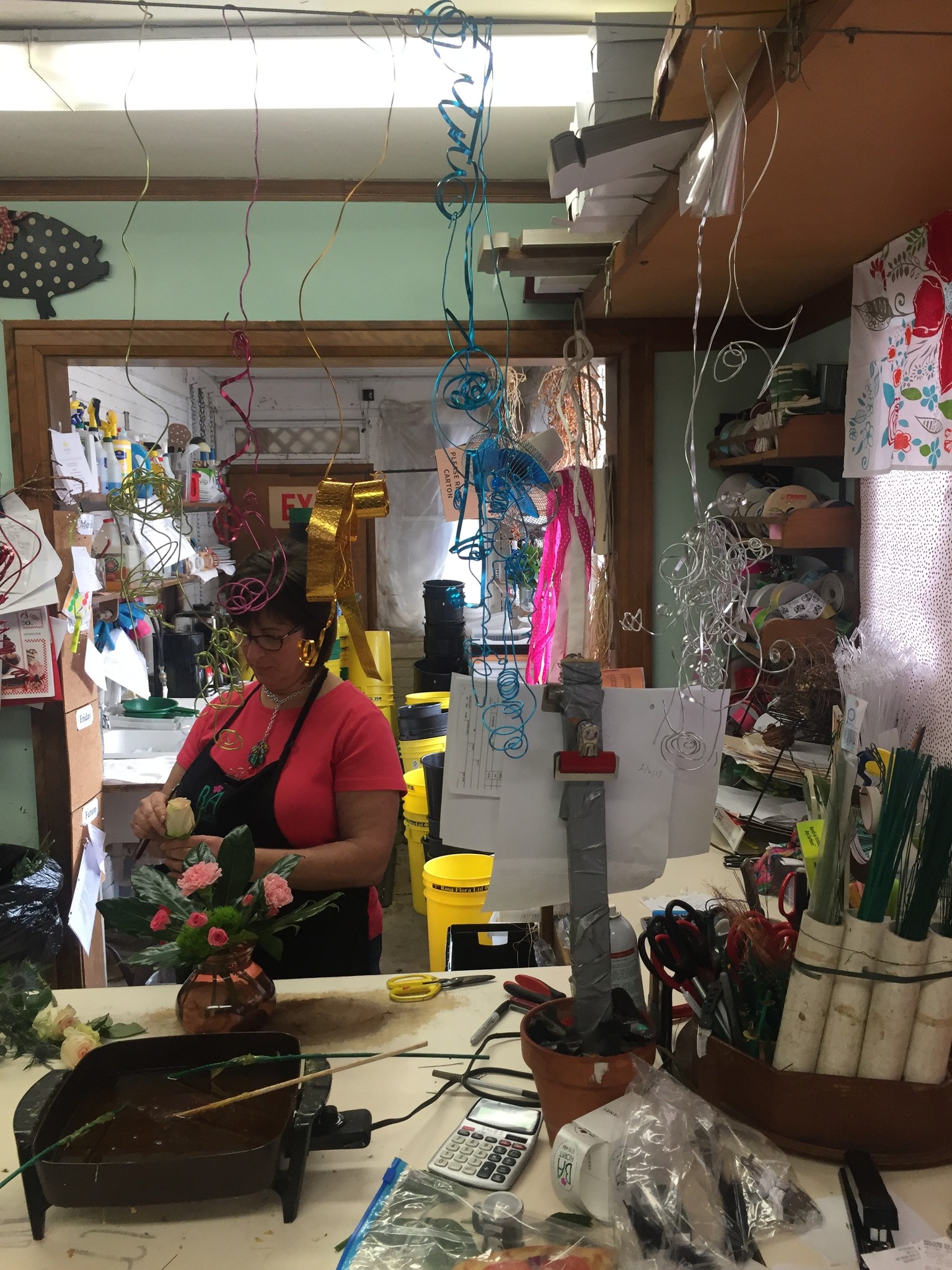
xmin=73 ymin=488 xmax=230 ymax=605
xmin=708 ymin=409 xmax=858 ymax=666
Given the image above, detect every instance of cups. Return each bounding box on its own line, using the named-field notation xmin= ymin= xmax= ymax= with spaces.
xmin=780 ymin=872 xmax=810 ymax=932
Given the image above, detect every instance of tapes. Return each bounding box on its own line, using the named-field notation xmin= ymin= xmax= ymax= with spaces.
xmin=860 ymin=786 xmax=883 ymax=834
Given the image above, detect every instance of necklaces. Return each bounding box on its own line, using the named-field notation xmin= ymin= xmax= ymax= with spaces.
xmin=247 ymin=673 xmax=321 ymax=768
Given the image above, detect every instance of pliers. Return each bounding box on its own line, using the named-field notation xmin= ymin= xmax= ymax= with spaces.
xmin=504 ymin=974 xmax=567 ymax=1004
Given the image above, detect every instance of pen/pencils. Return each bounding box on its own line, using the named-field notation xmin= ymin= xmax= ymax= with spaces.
xmin=431 ymin=1068 xmax=539 ymax=1099
xmin=794 ymin=867 xmax=807 ymax=932
xmin=471 ymin=997 xmax=510 ymax=1044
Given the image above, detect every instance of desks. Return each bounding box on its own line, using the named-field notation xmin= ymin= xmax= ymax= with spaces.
xmin=0 ymin=967 xmax=952 ymax=1270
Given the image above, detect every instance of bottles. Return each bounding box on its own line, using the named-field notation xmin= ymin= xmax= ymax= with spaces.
xmin=607 ymin=904 xmax=645 ymax=1012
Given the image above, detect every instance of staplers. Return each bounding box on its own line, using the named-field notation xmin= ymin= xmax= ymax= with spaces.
xmin=840 ymin=1150 xmax=900 ymax=1269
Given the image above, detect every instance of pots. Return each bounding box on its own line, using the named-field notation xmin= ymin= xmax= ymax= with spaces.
xmin=11 ymin=1032 xmax=371 ymax=1241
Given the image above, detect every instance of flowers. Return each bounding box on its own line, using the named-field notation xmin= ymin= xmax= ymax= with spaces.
xmin=0 ymin=959 xmax=148 ymax=1071
xmin=95 ymin=824 xmax=347 ymax=1025
xmin=166 ymin=797 xmax=196 ymax=839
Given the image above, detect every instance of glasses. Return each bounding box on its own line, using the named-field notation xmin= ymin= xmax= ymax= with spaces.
xmin=228 ymin=619 xmax=304 ymax=651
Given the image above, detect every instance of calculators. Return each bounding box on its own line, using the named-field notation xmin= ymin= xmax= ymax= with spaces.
xmin=427 ymin=1099 xmax=544 ymax=1192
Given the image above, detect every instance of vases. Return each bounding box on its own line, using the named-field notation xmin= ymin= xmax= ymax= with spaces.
xmin=175 ymin=939 xmax=276 ymax=1032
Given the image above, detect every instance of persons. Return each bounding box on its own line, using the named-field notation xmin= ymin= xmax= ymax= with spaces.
xmin=129 ymin=547 xmax=407 ymax=979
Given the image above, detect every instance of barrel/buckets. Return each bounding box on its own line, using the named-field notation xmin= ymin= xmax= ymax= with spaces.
xmin=327 ymin=581 xmax=494 ymax=973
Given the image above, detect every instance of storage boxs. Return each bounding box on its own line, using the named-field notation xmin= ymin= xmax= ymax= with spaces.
xmin=677 ymin=1021 xmax=952 ymax=1172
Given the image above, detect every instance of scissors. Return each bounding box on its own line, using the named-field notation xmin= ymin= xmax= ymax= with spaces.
xmin=636 ymin=867 xmax=808 ymax=1064
xmin=723 ymin=853 xmax=761 ymax=868
xmin=386 ymin=972 xmax=496 ymax=1003
